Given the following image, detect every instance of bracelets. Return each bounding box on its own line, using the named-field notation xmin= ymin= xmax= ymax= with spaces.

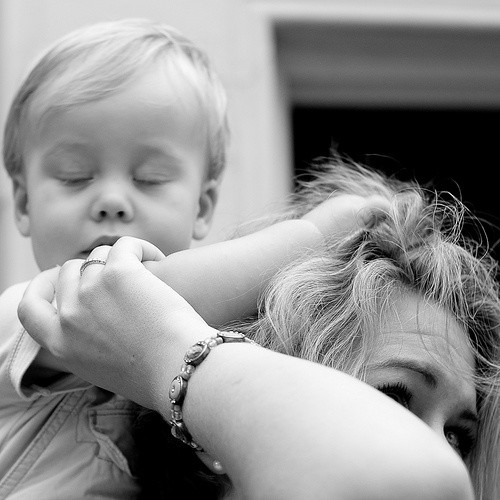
xmin=170 ymin=330 xmax=255 ymax=452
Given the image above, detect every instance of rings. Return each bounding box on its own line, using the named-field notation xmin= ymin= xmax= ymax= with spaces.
xmin=78 ymin=258 xmax=107 ymax=275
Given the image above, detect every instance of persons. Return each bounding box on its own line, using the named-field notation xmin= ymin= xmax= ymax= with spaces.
xmin=1 ymin=19 xmax=391 ymax=500
xmin=17 ymin=169 xmax=500 ymax=500
